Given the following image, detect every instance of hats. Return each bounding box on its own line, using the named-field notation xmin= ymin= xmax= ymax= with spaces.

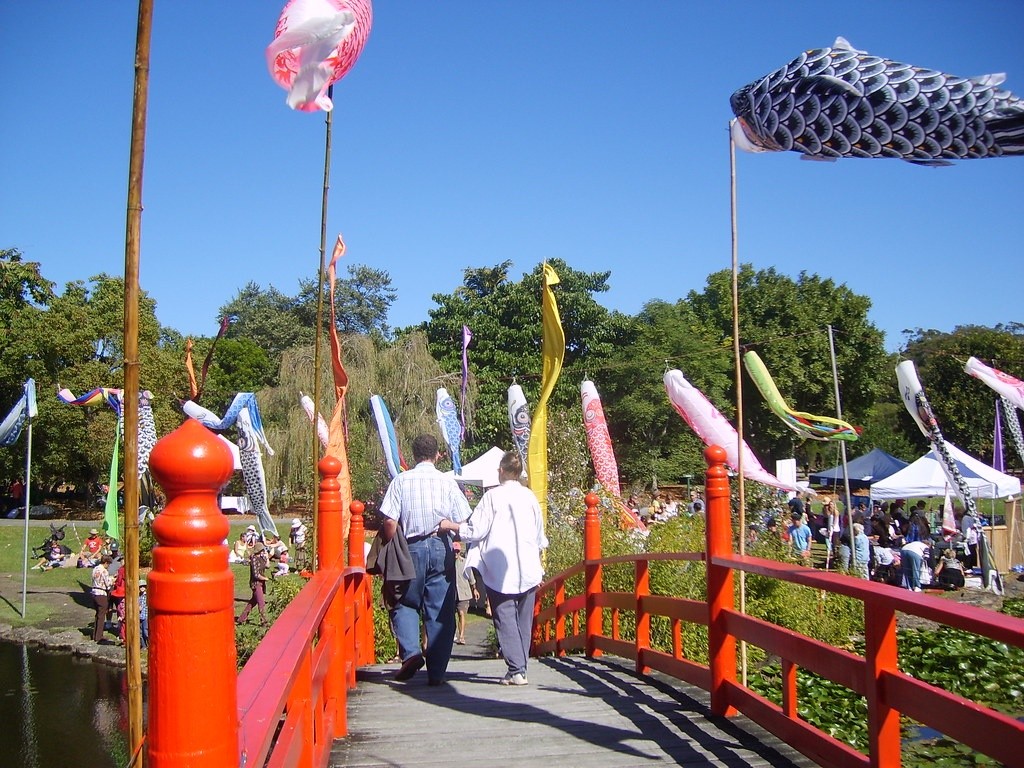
xmin=89 ymin=529 xmax=99 ymax=534
xmin=139 ymin=580 xmax=147 ymax=586
xmin=111 ymin=542 xmax=120 ymax=551
xmin=291 ymin=518 xmax=302 ymax=528
xmin=281 ymin=547 xmax=288 ymax=554
xmin=246 ymin=525 xmax=255 ymax=530
xmin=253 ymin=542 xmax=265 ymax=554
xmin=821 ymin=497 xmax=831 ymax=505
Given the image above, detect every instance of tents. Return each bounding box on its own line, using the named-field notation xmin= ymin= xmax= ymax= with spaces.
xmin=809 ymin=447 xmax=913 ymax=512
xmin=870 ymin=440 xmax=1021 ymax=569
xmin=442 ymin=446 xmax=550 ymax=496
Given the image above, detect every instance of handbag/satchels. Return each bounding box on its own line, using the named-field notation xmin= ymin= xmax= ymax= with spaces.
xmin=920 ymin=559 xmax=931 ymax=584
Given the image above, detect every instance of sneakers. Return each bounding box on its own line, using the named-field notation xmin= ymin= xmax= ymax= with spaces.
xmin=500 ymin=672 xmax=528 ymax=685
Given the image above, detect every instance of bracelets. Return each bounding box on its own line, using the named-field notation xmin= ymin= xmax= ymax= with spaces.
xmin=473 ymin=587 xmax=476 ymax=590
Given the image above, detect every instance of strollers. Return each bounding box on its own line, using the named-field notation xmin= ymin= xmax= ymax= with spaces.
xmin=29 ymin=522 xmax=72 ymax=560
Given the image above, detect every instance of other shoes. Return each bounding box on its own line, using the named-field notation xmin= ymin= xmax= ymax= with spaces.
xmin=430 ymin=678 xmax=446 ymax=686
xmin=41 ymin=564 xmax=46 ymax=572
xmin=453 ymin=636 xmax=465 ymax=644
xmin=31 ymin=565 xmax=40 ymax=569
xmin=394 ymin=654 xmax=425 ymax=680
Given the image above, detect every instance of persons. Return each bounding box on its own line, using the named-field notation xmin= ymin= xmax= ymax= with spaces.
xmin=7 ymin=478 xmax=23 ymax=508
xmin=30 ymin=517 xmax=308 ymax=648
xmin=379 ymin=434 xmax=472 ymax=684
xmin=570 ymin=489 xmax=984 ymax=590
xmin=379 ymin=541 xmax=480 ymax=664
xmin=439 ymin=453 xmax=551 ymax=686
